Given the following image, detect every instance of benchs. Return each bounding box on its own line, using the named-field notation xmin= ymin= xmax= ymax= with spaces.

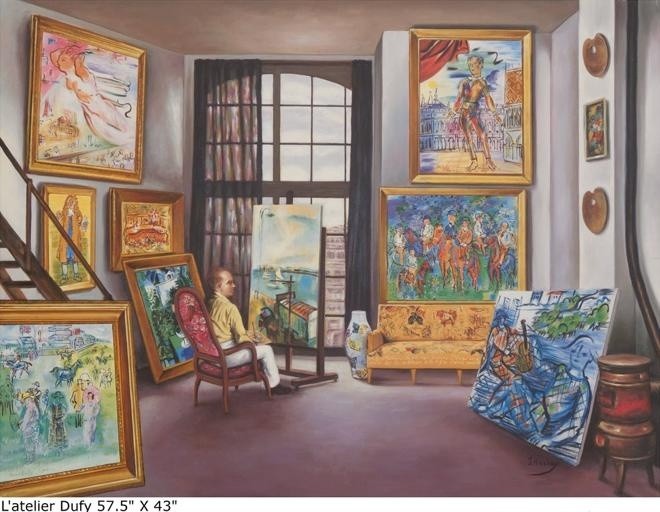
xmin=365 ymin=303 xmax=500 ymax=386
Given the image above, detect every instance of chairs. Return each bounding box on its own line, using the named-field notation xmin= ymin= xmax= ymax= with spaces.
xmin=169 ymin=285 xmax=279 ymax=420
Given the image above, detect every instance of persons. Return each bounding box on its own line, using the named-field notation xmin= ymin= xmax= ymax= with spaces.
xmin=204 ymin=266 xmax=292 ymax=395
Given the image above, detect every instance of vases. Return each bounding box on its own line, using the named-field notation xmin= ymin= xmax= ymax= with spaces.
xmin=343 ymin=308 xmax=374 ymax=381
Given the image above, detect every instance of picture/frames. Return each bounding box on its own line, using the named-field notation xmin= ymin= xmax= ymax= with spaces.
xmin=36 ymin=182 xmax=98 ymax=295
xmin=376 ymin=185 xmax=529 ymax=305
xmin=1 ymin=297 xmax=147 ymax=496
xmin=583 ymin=97 xmax=611 ymax=163
xmin=22 ymin=10 xmax=151 ymax=187
xmin=105 ymin=186 xmax=187 ymax=275
xmin=405 ymin=24 xmax=538 ymax=189
xmin=120 ymin=252 xmax=209 ymax=385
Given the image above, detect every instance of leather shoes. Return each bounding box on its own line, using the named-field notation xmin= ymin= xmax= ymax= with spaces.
xmin=269 ymin=382 xmax=291 ymax=397
